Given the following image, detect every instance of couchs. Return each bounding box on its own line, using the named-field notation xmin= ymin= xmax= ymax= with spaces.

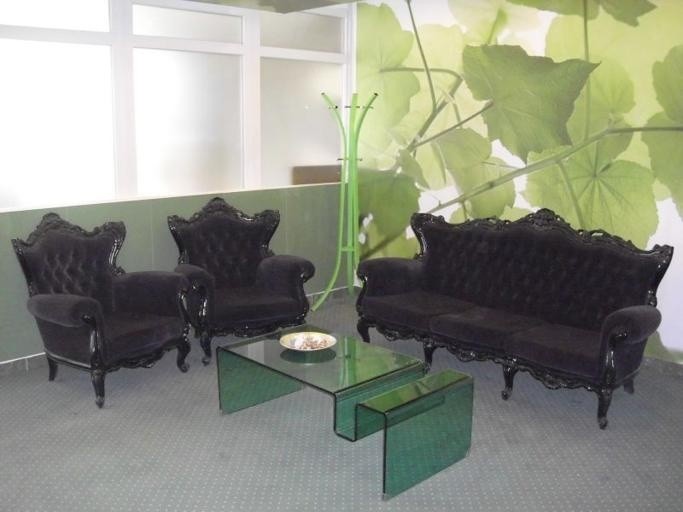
xmin=355 ymin=207 xmax=674 ymax=430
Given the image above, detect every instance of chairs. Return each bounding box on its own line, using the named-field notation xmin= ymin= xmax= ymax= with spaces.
xmin=165 ymin=195 xmax=316 ymax=366
xmin=8 ymin=212 xmax=191 ymax=407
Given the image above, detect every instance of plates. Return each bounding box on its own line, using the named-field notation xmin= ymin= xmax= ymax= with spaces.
xmin=279 ymin=330 xmax=337 ymax=352
xmin=279 ymin=349 xmax=335 ymax=363
